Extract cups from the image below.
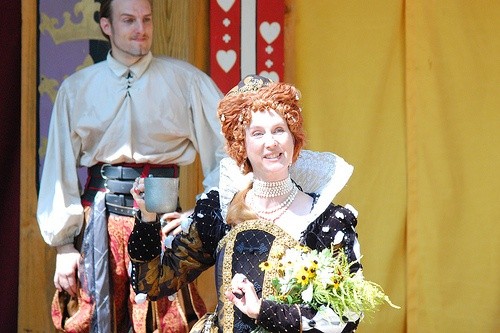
[145,178,179,212]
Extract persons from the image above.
[36,0,228,333]
[128,74,364,333]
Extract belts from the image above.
[86,190,135,207]
[88,164,174,178]
[89,178,135,192]
[106,203,136,216]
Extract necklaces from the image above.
[247,173,299,222]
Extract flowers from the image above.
[259,245,401,325]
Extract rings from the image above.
[242,276,248,283]
[134,176,141,194]
